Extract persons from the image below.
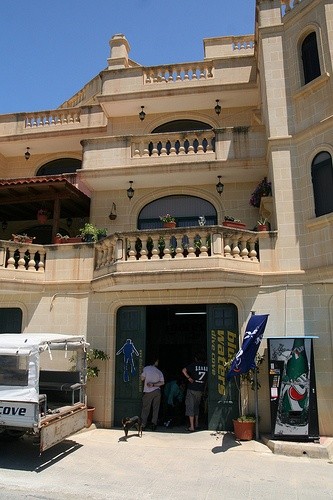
[182,355,208,431]
[141,356,164,430]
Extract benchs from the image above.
[39,381,83,406]
[39,394,46,417]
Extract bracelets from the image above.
[152,384,154,387]
[187,377,191,379]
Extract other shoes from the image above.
[152,425,156,431]
[142,427,145,431]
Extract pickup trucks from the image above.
[0,332,89,454]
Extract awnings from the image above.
[0,178,91,244]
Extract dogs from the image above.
[122,416,144,438]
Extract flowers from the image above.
[249,177,271,208]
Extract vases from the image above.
[109,214,117,220]
[38,215,47,224]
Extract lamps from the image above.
[24,146,31,161]
[66,217,73,227]
[126,181,134,201]
[139,106,146,122]
[215,176,224,197]
[214,99,221,116]
[1,220,8,232]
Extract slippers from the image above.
[185,427,195,432]
[195,426,201,429]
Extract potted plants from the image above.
[158,214,175,222]
[222,215,246,228]
[69,347,111,427]
[224,350,266,441]
[37,208,50,219]
[11,233,36,243]
[256,211,272,231]
[61,234,83,243]
[79,223,95,242]
[95,228,109,240]
[53,233,63,244]
[163,222,176,228]
